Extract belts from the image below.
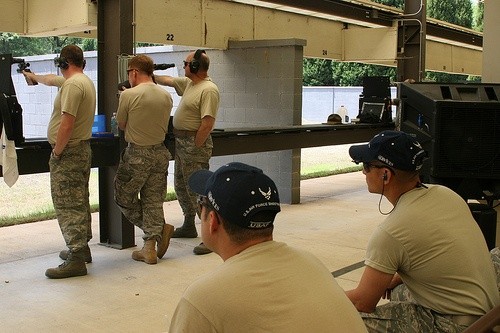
[51,142,82,148]
[442,314,483,327]
[128,142,161,149]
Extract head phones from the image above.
[189,49,206,74]
[54,56,86,69]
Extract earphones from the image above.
[384,173,387,181]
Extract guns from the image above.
[16,62,38,86]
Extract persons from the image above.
[22,45,96,278]
[344,131,500,333]
[153,49,220,254]
[170,162,370,333]
[113,55,174,265]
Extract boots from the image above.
[193,242,213,255]
[45,252,87,279]
[157,224,174,259]
[170,216,198,238]
[59,244,92,264]
[132,240,157,264]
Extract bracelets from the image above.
[53,150,60,158]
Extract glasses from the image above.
[125,68,139,75]
[196,195,222,224]
[182,60,191,67]
[363,162,395,176]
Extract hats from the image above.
[189,162,281,229]
[349,130,425,172]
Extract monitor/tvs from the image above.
[396,82,500,202]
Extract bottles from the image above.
[338,105,351,124]
[110,112,118,136]
